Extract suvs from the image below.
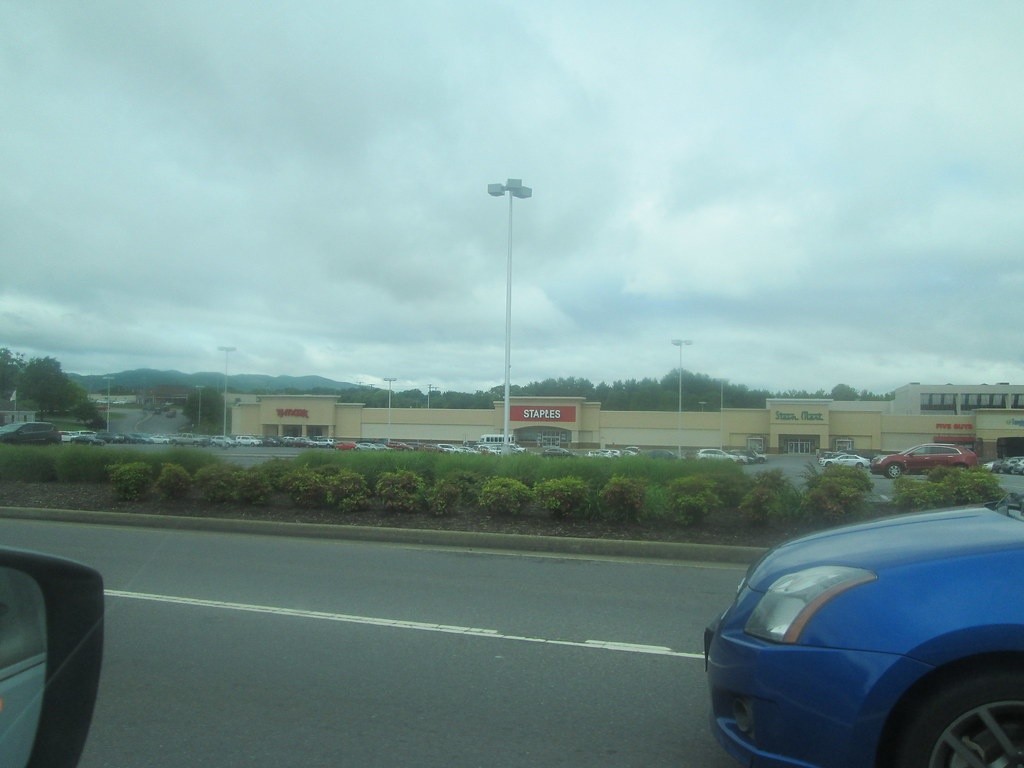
[0,422,62,446]
[869,442,981,479]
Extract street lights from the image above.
[486,177,533,454]
[383,377,397,443]
[102,375,115,435]
[671,339,693,457]
[217,345,236,436]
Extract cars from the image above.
[816,449,871,470]
[61,429,768,465]
[154,404,177,418]
[702,493,1024,768]
[982,455,1024,476]
[96,398,127,406]
